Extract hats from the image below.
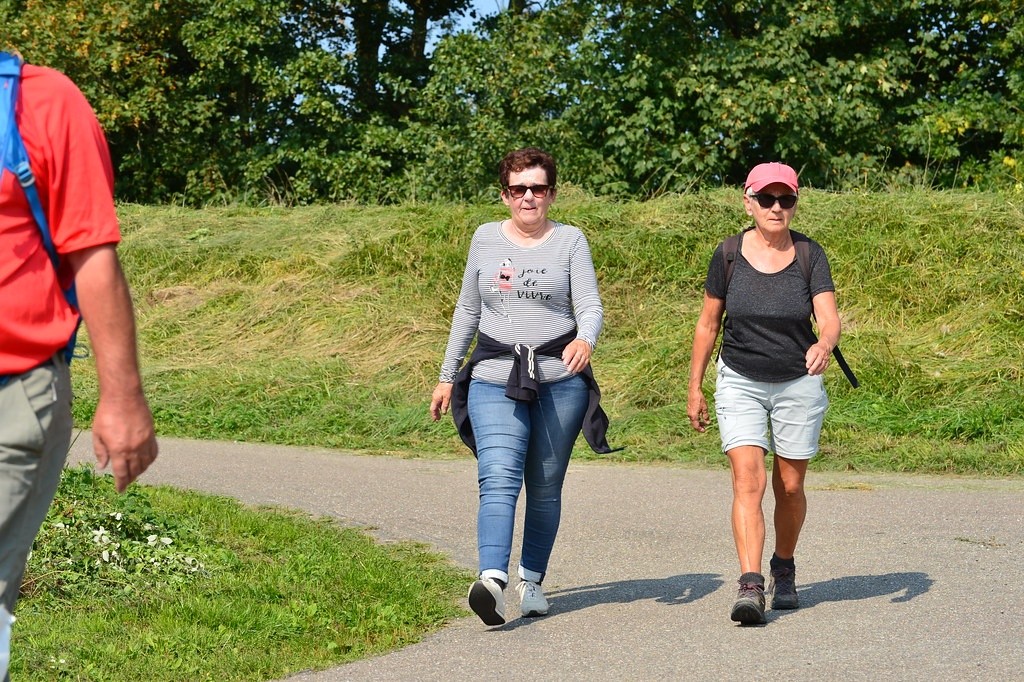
[744,163,799,198]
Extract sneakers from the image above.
[730,580,766,624]
[769,567,800,610]
[466,579,505,627]
[515,580,548,619]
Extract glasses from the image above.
[504,184,552,198]
[749,193,798,208]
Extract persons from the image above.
[431,146,604,627]
[686,162,841,624]
[0,50,157,682]
